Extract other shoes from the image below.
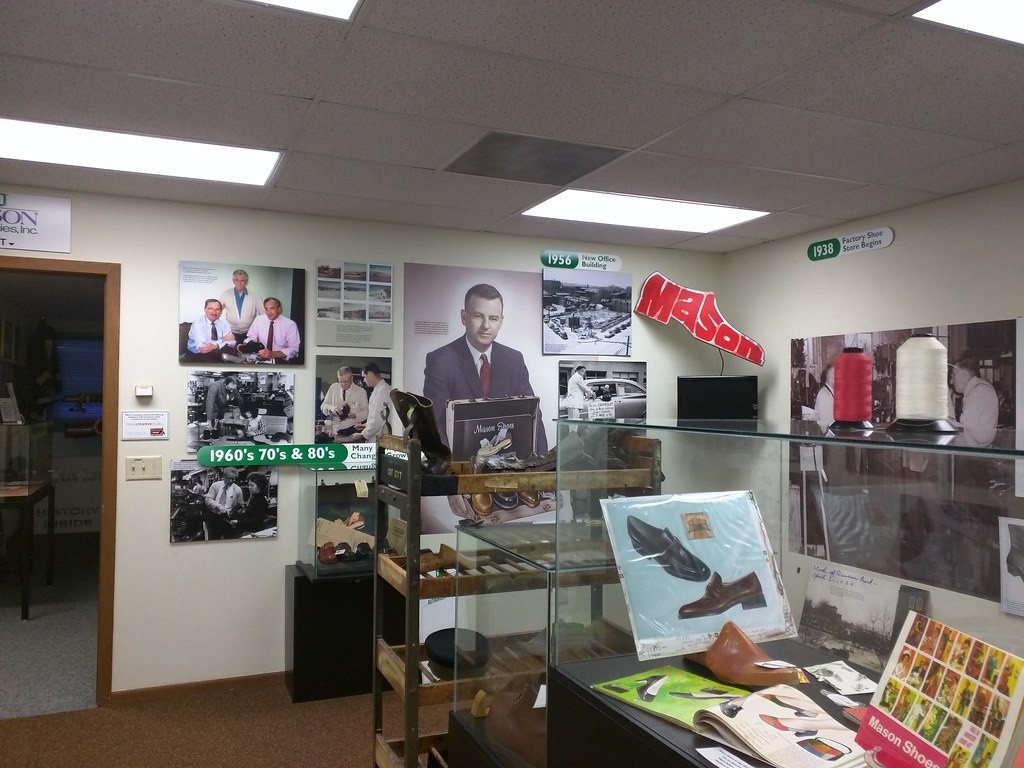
[476,432,513,457]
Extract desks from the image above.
[315,430,365,443]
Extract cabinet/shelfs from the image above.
[372,432,665,767]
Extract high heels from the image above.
[635,675,669,702]
[669,688,753,719]
[755,693,818,719]
[759,714,818,737]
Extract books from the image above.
[590,664,867,768]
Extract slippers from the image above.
[521,451,555,467]
[485,451,527,470]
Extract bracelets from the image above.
[217,343,219,350]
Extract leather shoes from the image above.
[518,490,541,508]
[490,491,521,510]
[318,542,337,563]
[356,542,374,560]
[676,571,767,621]
[335,542,356,562]
[370,537,393,554]
[467,491,494,516]
[626,514,711,582]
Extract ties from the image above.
[479,354,492,397]
[266,321,274,351]
[218,486,227,508]
[210,322,217,341]
[342,389,346,401]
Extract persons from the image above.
[236,297,301,364]
[231,403,267,437]
[351,361,392,443]
[224,472,270,539]
[184,298,236,364]
[597,384,612,401]
[815,361,863,495]
[422,284,549,518]
[949,355,1000,535]
[203,466,245,540]
[193,480,207,495]
[563,366,596,433]
[320,366,368,434]
[200,376,246,430]
[217,270,266,345]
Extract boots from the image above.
[1006,524,1024,582]
[575,424,633,469]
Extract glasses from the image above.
[206,307,221,313]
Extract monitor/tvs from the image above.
[678,375,758,420]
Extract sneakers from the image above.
[334,511,365,530]
